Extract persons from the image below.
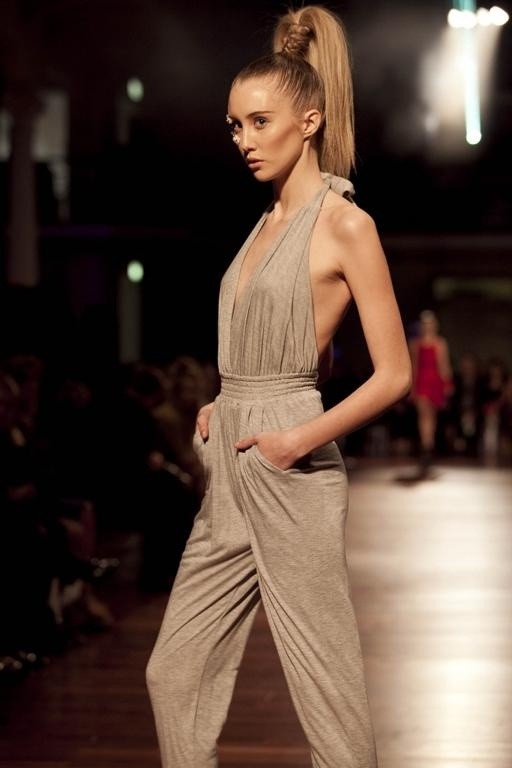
[344,308,511,465]
[1,351,220,672]
[145,5,413,768]
